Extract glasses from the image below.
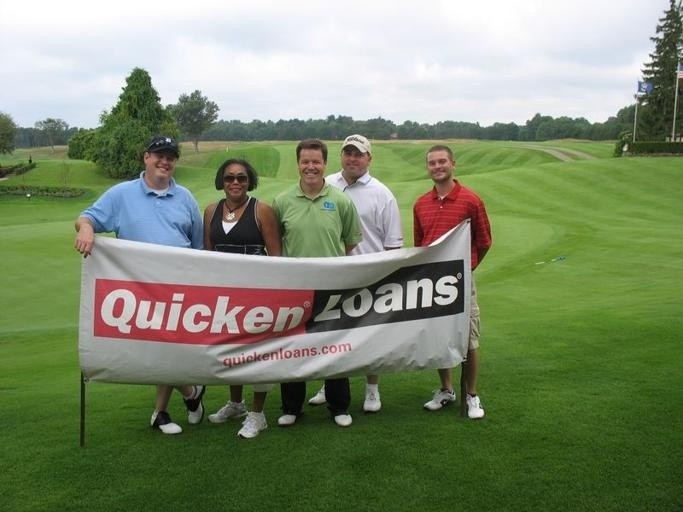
[223,175,247,183]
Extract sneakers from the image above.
[308,384,327,405]
[424,389,456,411]
[334,414,352,426]
[208,399,249,423]
[363,391,381,412]
[466,393,485,419]
[183,384,206,425]
[277,414,297,425]
[150,410,183,434]
[237,410,268,439]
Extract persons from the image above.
[412,144,491,419]
[203,158,281,439]
[307,134,402,413]
[73,136,206,434]
[272,140,362,426]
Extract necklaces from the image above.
[224,196,248,221]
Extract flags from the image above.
[638,81,653,93]
[678,64,683,79]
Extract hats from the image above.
[146,135,178,156]
[340,134,370,155]
